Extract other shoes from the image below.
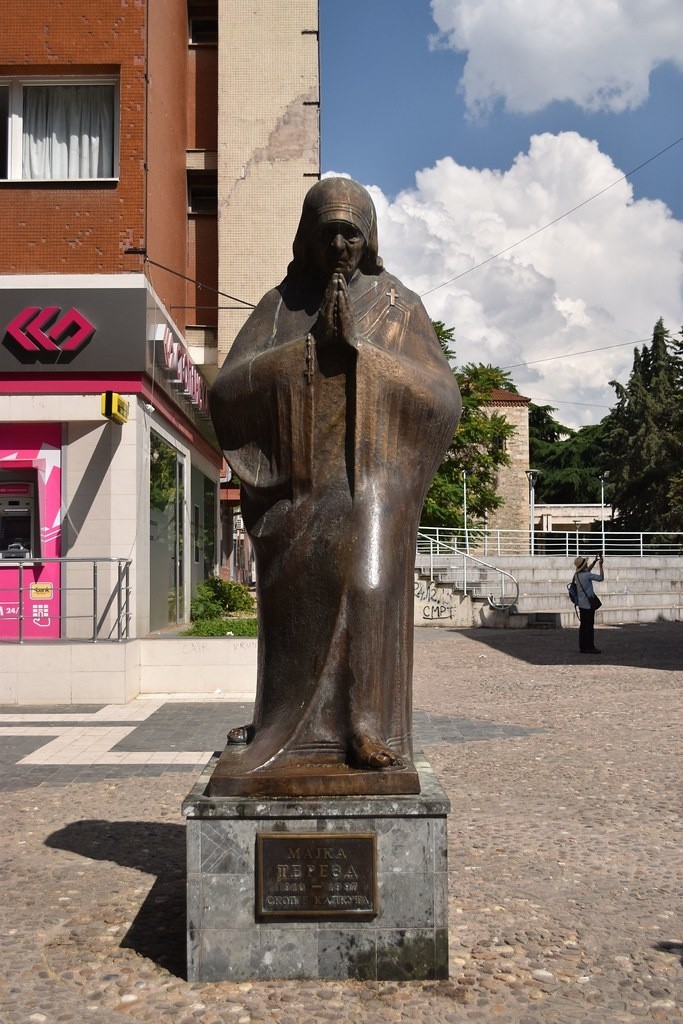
[580,645,601,654]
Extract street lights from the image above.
[524,469,541,556]
[461,469,470,555]
[599,471,611,557]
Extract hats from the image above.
[574,556,589,572]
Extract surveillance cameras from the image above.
[147,405,155,413]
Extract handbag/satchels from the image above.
[577,572,603,610]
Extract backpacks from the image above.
[566,574,578,606]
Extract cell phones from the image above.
[599,553,602,558]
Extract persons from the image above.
[570,552,602,653]
[200,178,462,770]
